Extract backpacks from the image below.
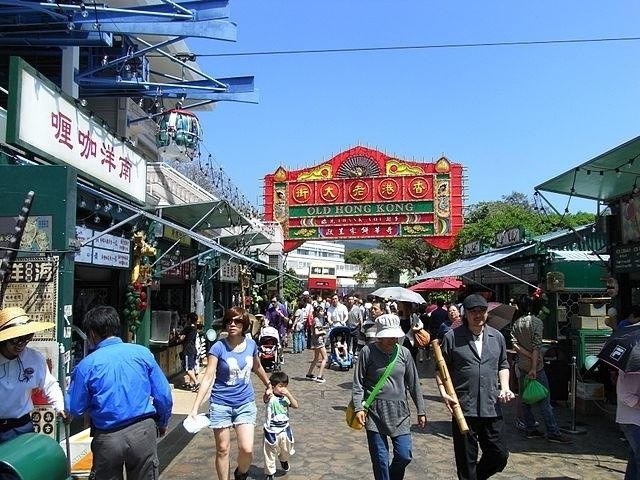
[509,363,519,394]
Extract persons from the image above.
[194,333,202,377]
[352,302,435,366]
[290,290,353,384]
[613,371,640,480]
[264,296,289,365]
[604,308,640,444]
[263,372,298,480]
[508,298,577,445]
[0,306,74,443]
[65,305,172,480]
[272,295,289,348]
[352,314,427,480]
[260,339,276,358]
[188,306,275,480]
[176,310,202,392]
[436,294,518,480]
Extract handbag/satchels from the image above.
[522,376,549,406]
[314,322,327,336]
[347,324,360,338]
[307,314,314,326]
[345,400,368,431]
[413,325,431,347]
[179,350,185,361]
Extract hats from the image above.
[1,306,57,342]
[374,313,405,339]
[463,294,489,309]
[302,290,310,296]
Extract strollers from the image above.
[324,326,352,371]
[259,326,284,371]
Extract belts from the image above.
[1,412,30,432]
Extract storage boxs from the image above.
[597,316,613,330]
[575,398,604,416]
[576,380,605,401]
[576,316,598,330]
[579,303,607,316]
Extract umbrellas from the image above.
[597,326,640,376]
[372,286,427,304]
[449,302,515,332]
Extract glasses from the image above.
[270,300,277,303]
[226,319,246,325]
[8,336,33,344]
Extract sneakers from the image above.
[513,418,539,429]
[526,429,547,437]
[306,374,316,381]
[279,355,284,364]
[192,383,200,391]
[280,461,291,472]
[316,375,326,383]
[265,474,275,480]
[182,384,194,390]
[548,434,573,444]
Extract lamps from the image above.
[116,204,123,214]
[156,85,163,96]
[205,220,212,228]
[564,245,568,251]
[81,6,89,18]
[586,171,592,181]
[533,207,538,215]
[130,68,139,80]
[94,214,101,224]
[100,52,109,67]
[114,71,124,84]
[571,241,578,250]
[591,226,596,233]
[107,199,113,212]
[217,207,223,214]
[569,228,574,234]
[632,185,639,193]
[79,196,86,208]
[130,223,139,233]
[564,209,570,217]
[628,159,634,169]
[89,112,96,125]
[570,188,577,195]
[103,201,108,213]
[125,62,131,72]
[92,22,102,30]
[534,192,539,198]
[587,261,593,267]
[599,171,604,181]
[95,200,102,211]
[175,247,181,256]
[80,95,88,106]
[540,207,545,214]
[138,97,144,109]
[615,167,622,179]
[100,120,107,135]
[152,241,159,247]
[582,235,586,242]
[109,219,116,227]
[154,98,161,107]
[175,97,185,107]
[66,14,76,30]
